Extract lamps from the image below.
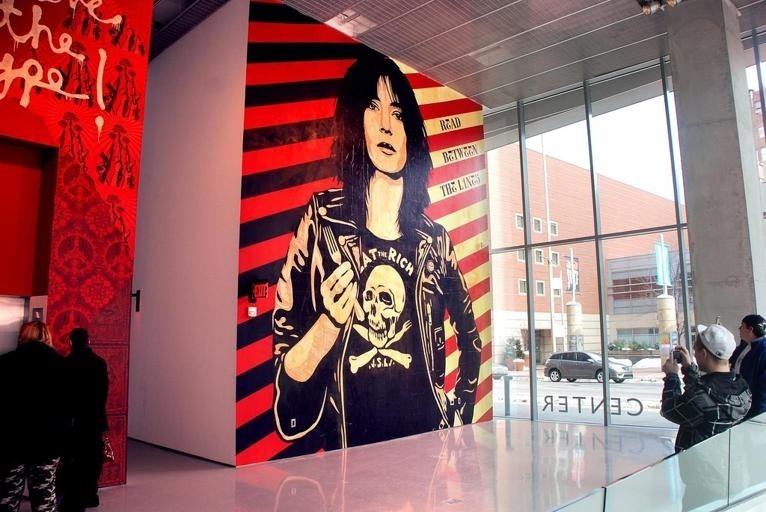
[635,0,683,17]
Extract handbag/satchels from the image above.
[102,437,115,463]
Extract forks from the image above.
[322,226,366,324]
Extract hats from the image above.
[697,324,736,360]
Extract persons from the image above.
[661,325,754,452]
[729,315,766,418]
[56,329,108,512]
[0,320,69,512]
[273,56,481,453]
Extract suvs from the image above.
[542,350,632,384]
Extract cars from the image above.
[492,362,508,381]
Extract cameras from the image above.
[670,350,682,363]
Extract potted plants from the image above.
[511,335,525,372]
[503,334,515,371]
